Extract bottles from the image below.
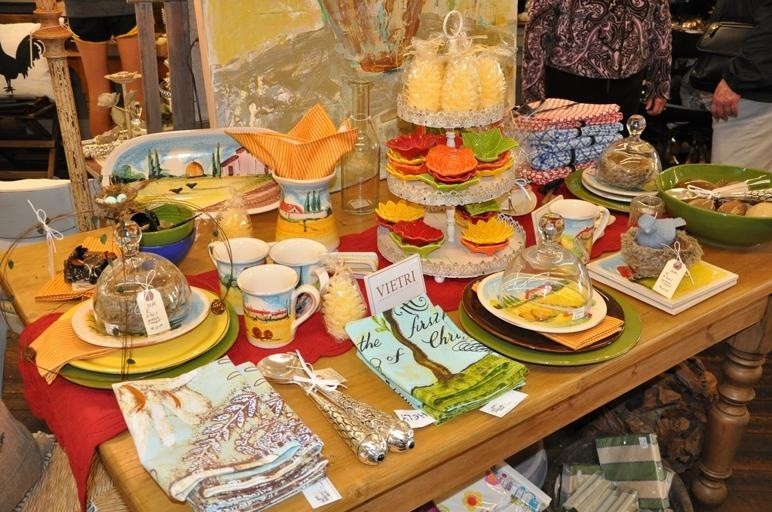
[340,79,380,216]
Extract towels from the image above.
[227,104,360,180]
[515,96,625,186]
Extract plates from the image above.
[510,179,526,213]
[459,270,644,367]
[384,127,520,193]
[564,163,665,213]
[374,200,445,258]
[57,286,240,390]
[454,199,515,256]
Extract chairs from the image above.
[0,0,60,181]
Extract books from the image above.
[430,429,677,512]
[585,250,741,310]
[585,270,739,318]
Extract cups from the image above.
[548,199,610,266]
[499,189,537,216]
[626,195,664,230]
[207,237,331,349]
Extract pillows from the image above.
[0,23,56,103]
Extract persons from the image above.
[707,0,771,176]
[518,0,674,149]
[61,1,149,140]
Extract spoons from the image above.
[258,352,415,465]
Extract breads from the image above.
[676,179,772,217]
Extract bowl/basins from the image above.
[130,203,196,268]
[656,163,772,249]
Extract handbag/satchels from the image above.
[688,21,757,94]
